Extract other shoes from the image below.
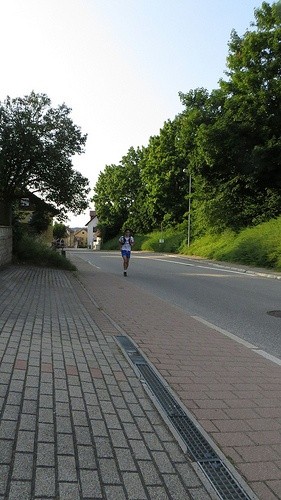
[123,271,127,277]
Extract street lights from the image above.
[183,168,192,248]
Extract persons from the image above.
[119,229,135,276]
